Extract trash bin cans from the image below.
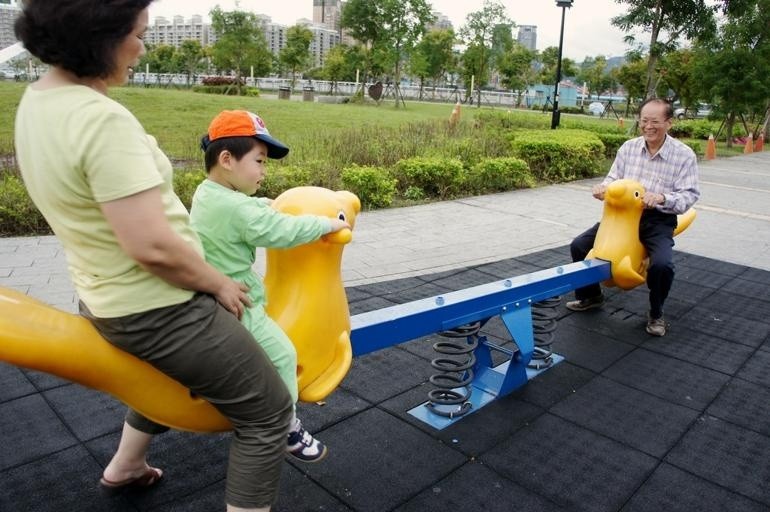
[278,86,291,99]
[303,87,314,101]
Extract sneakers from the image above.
[566,293,606,312]
[645,305,666,337]
[284,417,328,464]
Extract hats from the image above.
[199,109,289,160]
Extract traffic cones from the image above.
[455,101,462,118]
[742,131,754,154]
[754,131,764,152]
[704,133,718,160]
[448,110,459,122]
[618,116,624,128]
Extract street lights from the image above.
[551,0,576,131]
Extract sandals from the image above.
[99,466,164,498]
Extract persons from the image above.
[186,108,352,463]
[9,0,295,511]
[564,97,701,338]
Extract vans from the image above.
[674,102,720,120]
